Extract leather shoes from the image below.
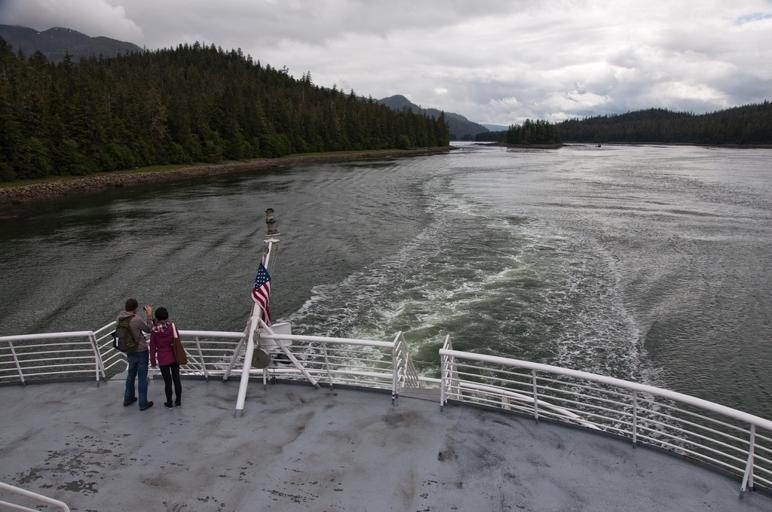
[123,397,137,406]
[140,400,153,411]
[164,402,172,408]
[175,401,180,406]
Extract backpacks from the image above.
[112,315,140,352]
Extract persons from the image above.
[149,306,183,408]
[115,298,156,411]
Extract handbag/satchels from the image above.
[172,337,188,364]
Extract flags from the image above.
[249,264,275,327]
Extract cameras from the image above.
[143,307,146,311]
